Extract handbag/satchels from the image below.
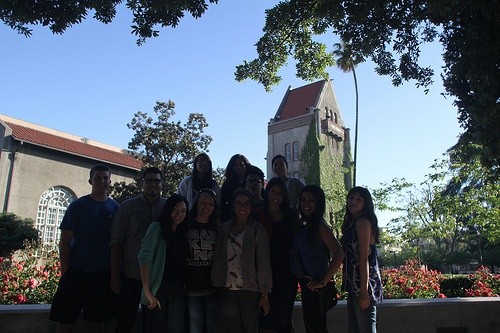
[318,284,337,313]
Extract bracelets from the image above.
[261,295,268,299]
[320,281,326,286]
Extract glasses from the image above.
[246,179,262,184]
[232,200,253,209]
[143,178,162,184]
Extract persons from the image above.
[289,185,346,333]
[174,188,221,333]
[137,195,189,333]
[177,154,220,212]
[210,187,273,333]
[272,155,308,208]
[341,187,382,333]
[252,177,298,333]
[48,164,122,333]
[110,167,168,333]
[220,155,266,222]
